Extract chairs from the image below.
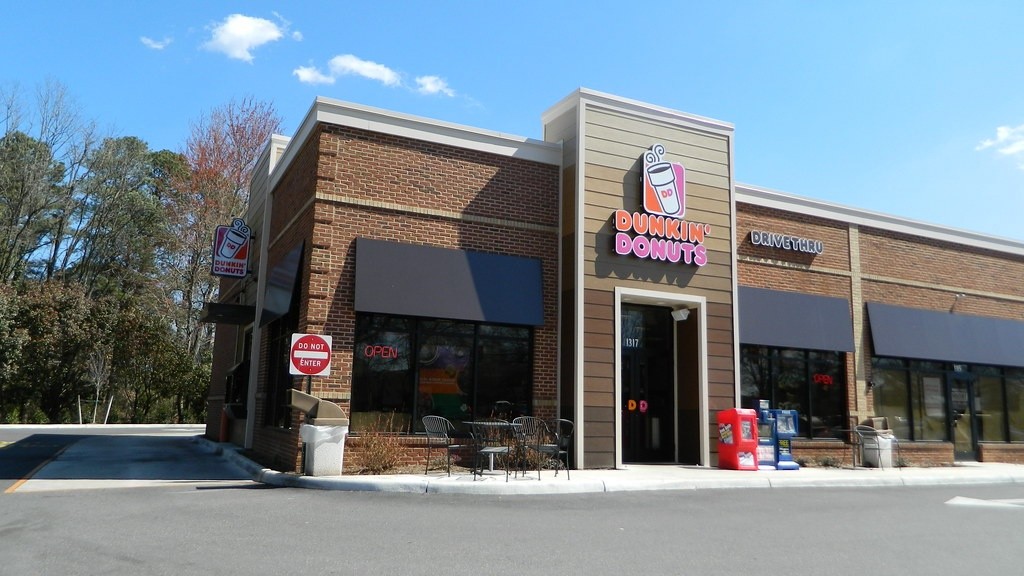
[421,415,476,478]
[522,417,575,481]
[835,414,869,470]
[470,417,521,482]
[512,415,559,477]
[853,425,903,471]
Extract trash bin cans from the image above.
[856,416,894,468]
[286,388,350,476]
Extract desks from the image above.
[461,421,523,476]
[838,428,893,471]
